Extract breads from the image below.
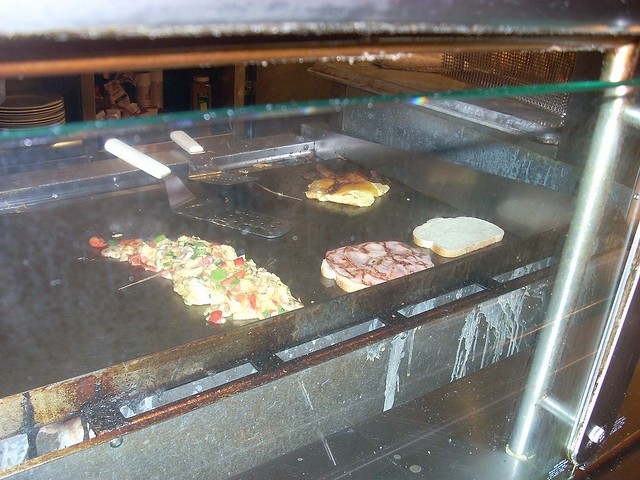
[306,177,389,207]
[413,215,505,258]
[320,240,435,292]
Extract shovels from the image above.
[169,130,261,184]
[104,139,293,240]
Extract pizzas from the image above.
[87,231,305,326]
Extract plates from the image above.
[0,94,64,131]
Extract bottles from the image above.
[190,75,213,111]
[134,72,152,107]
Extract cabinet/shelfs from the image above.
[0,64,245,175]
